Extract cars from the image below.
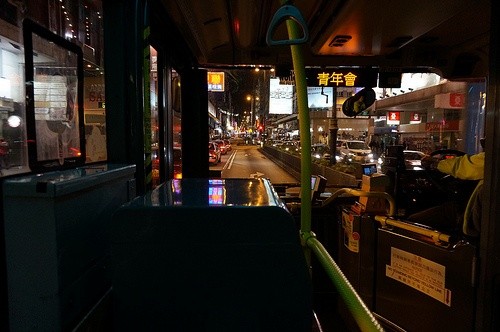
[243,133,427,175]
[148,131,237,179]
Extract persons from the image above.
[420,136,486,248]
[0,137,10,170]
[370,136,445,155]
